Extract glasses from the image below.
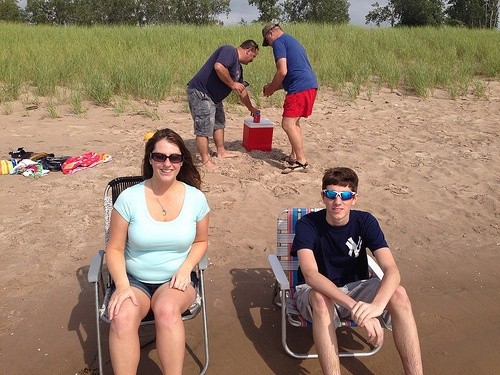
[150,152,184,163]
[323,189,357,201]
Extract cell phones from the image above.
[241,81,249,87]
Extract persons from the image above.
[187,40,260,172]
[262,22,318,174]
[290,166,424,375]
[105,127,211,375]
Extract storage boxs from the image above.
[242,118,274,152]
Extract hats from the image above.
[261,22,279,47]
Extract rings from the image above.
[184,285,187,288]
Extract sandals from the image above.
[282,162,308,174]
[282,155,297,165]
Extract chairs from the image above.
[87,177,209,375]
[268,207,385,359]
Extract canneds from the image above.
[254,110,260,123]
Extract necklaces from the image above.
[151,179,175,215]
